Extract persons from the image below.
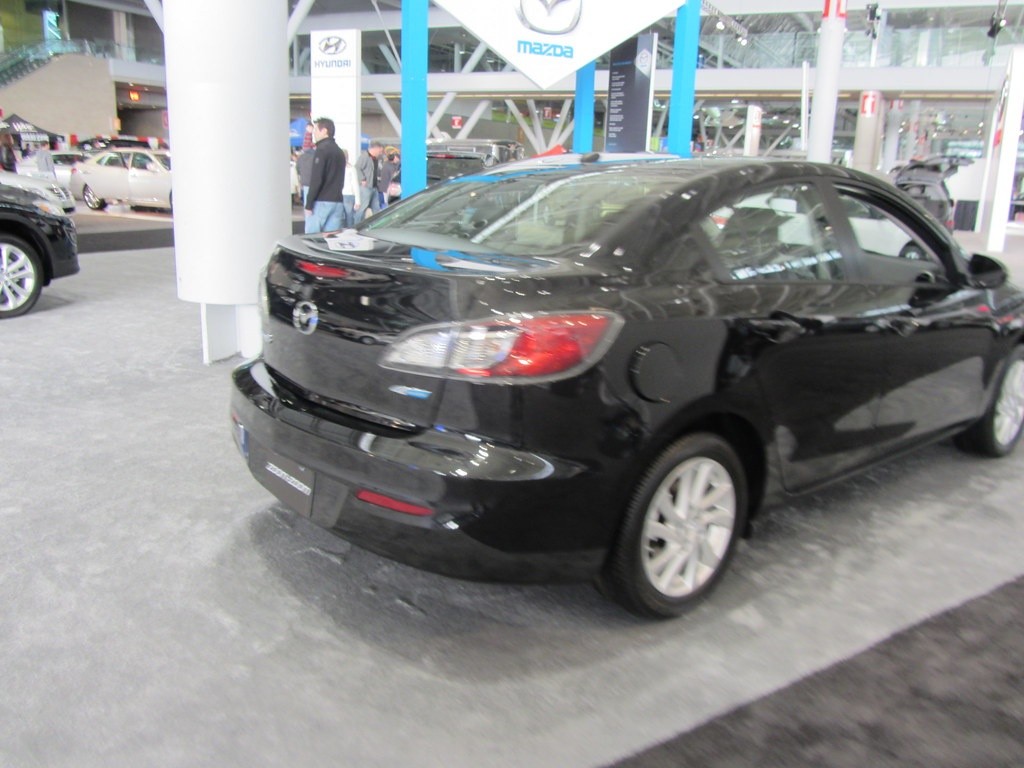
[24,144,30,154]
[380,153,400,205]
[36,140,57,183]
[354,139,382,223]
[304,118,346,234]
[0,129,17,173]
[289,144,316,204]
[341,150,360,227]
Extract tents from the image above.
[290,117,371,149]
[2,114,65,143]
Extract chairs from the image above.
[716,207,816,281]
[516,220,576,254]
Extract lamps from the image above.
[988,16,1004,38]
[866,4,882,38]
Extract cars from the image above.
[0,168,76,214]
[0,180,80,318]
[230,153,1024,619]
[71,147,173,210]
[387,139,524,206]
